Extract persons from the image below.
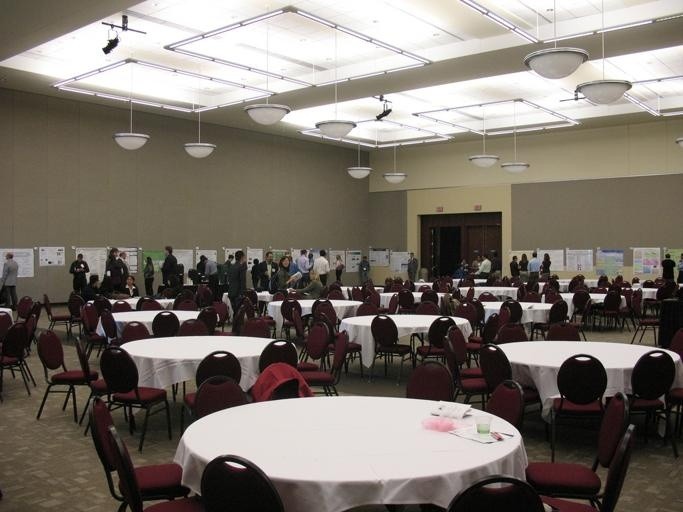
[196,247,344,318]
[359,255,370,286]
[676,253,682,284]
[143,247,179,298]
[1,254,18,311]
[69,247,140,300]
[407,253,418,283]
[457,254,499,280]
[660,253,675,280]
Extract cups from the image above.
[474,415,491,434]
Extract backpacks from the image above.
[268,273,278,294]
